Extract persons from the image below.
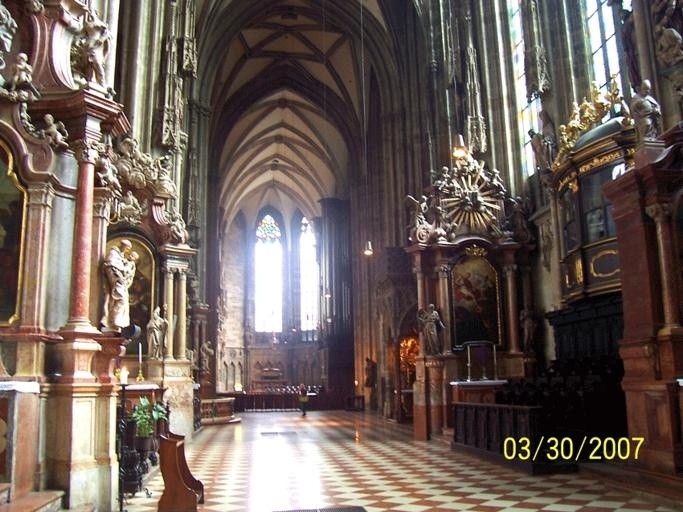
[146,308,170,360]
[81,9,110,87]
[519,303,538,351]
[631,80,664,141]
[298,383,309,416]
[526,82,603,174]
[422,304,447,354]
[10,54,68,152]
[189,277,212,371]
[455,272,490,310]
[100,240,139,332]
[406,156,528,240]
[97,136,188,240]
[653,24,683,69]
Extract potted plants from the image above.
[122,395,168,452]
